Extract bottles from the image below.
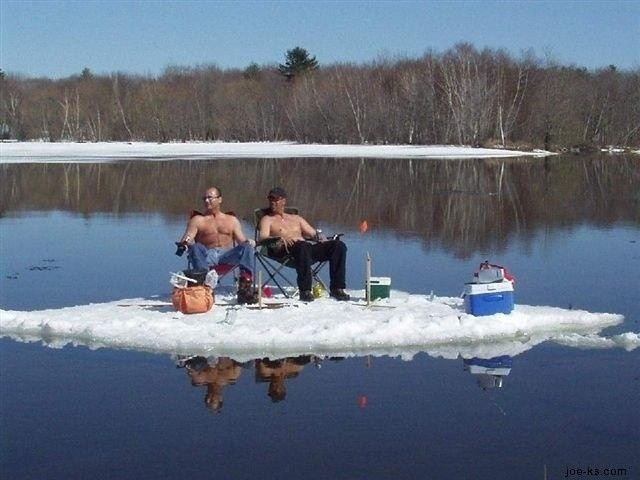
[313,280,323,299]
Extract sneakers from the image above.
[331,287,350,301]
[300,289,315,301]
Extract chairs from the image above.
[185,208,241,306]
[252,207,346,299]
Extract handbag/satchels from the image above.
[173,285,215,315]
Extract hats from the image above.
[267,187,288,199]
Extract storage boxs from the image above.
[464,281,515,317]
[463,352,512,379]
[365,276,392,301]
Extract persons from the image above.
[174,184,260,305]
[183,355,244,416]
[256,187,352,302]
[253,358,305,405]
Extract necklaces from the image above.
[278,215,288,224]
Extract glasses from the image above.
[202,195,219,201]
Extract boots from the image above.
[238,276,258,305]
